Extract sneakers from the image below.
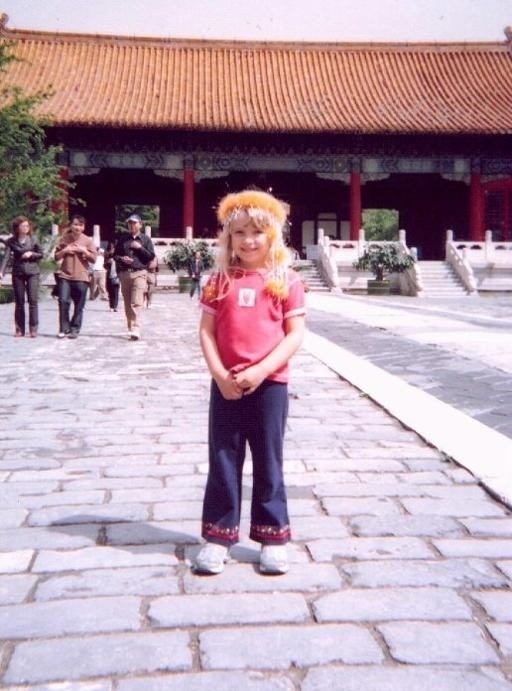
[30,331,37,337]
[260,543,289,574]
[58,333,66,338]
[14,330,24,337]
[190,292,201,300]
[109,308,117,312]
[68,331,78,338]
[127,331,140,340]
[142,300,152,309]
[195,542,231,573]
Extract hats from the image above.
[126,215,141,222]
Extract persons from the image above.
[194,190,306,577]
[49,235,159,312]
[188,250,204,299]
[0,216,43,337]
[55,214,97,339]
[113,215,156,342]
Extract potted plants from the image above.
[354,246,414,295]
[162,241,214,293]
